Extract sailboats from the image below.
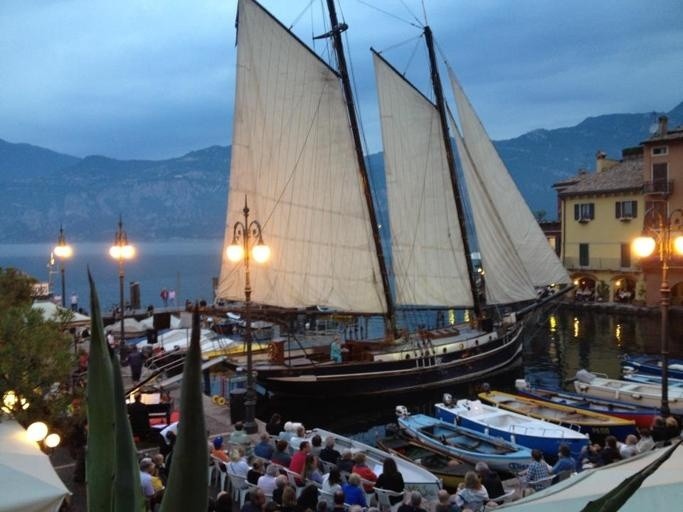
[215,0,578,404]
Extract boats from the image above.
[285,354,682,499]
[0,266,54,303]
[279,312,349,347]
[106,304,287,370]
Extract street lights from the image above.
[53,224,73,309]
[226,192,271,435]
[68,327,90,357]
[108,212,135,366]
[629,206,682,441]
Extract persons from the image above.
[524,428,656,494]
[138,413,405,511]
[53,285,208,387]
[576,284,637,304]
[128,392,151,449]
[330,333,350,363]
[647,415,682,442]
[398,461,505,511]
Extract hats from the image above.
[214,436,223,445]
